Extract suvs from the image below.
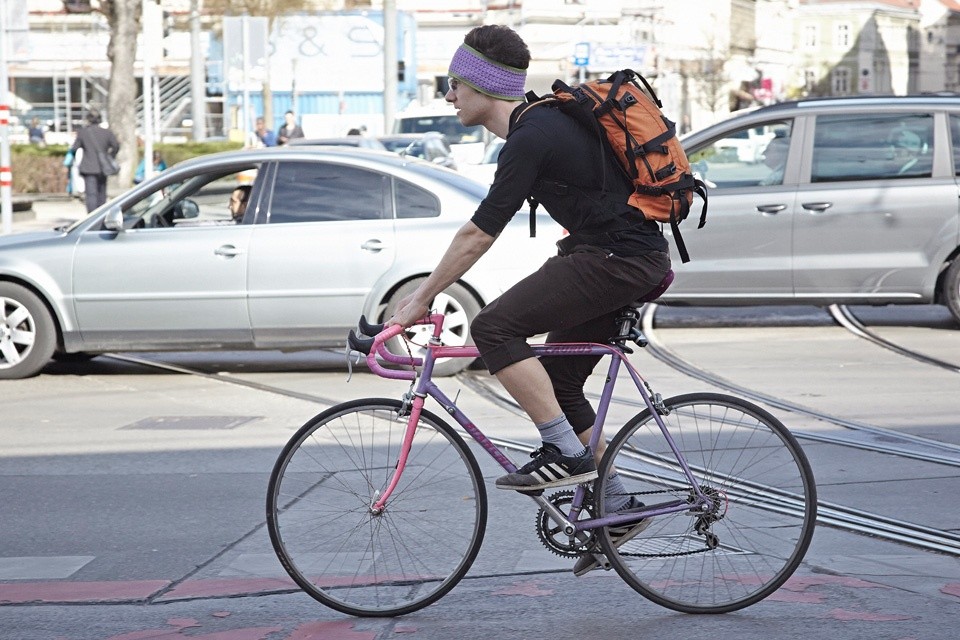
[651,90,960,326]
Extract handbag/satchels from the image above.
[96,152,120,177]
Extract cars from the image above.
[0,143,569,381]
[284,98,551,218]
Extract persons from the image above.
[228,185,252,224]
[890,129,926,174]
[132,150,166,184]
[346,129,387,151]
[382,24,671,578]
[758,136,788,186]
[28,117,44,144]
[70,109,120,214]
[253,117,276,147]
[729,80,754,112]
[277,111,304,145]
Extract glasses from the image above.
[447,77,465,93]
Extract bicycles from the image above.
[263,269,822,617]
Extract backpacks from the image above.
[517,66,707,264]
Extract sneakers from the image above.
[494,440,599,490]
[571,497,657,576]
